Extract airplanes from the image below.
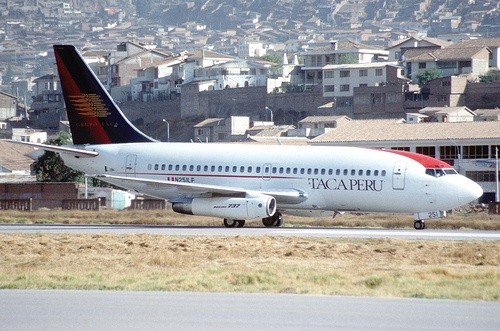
[0,43,486,231]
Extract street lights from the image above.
[265,106,273,121]
[161,119,171,142]
